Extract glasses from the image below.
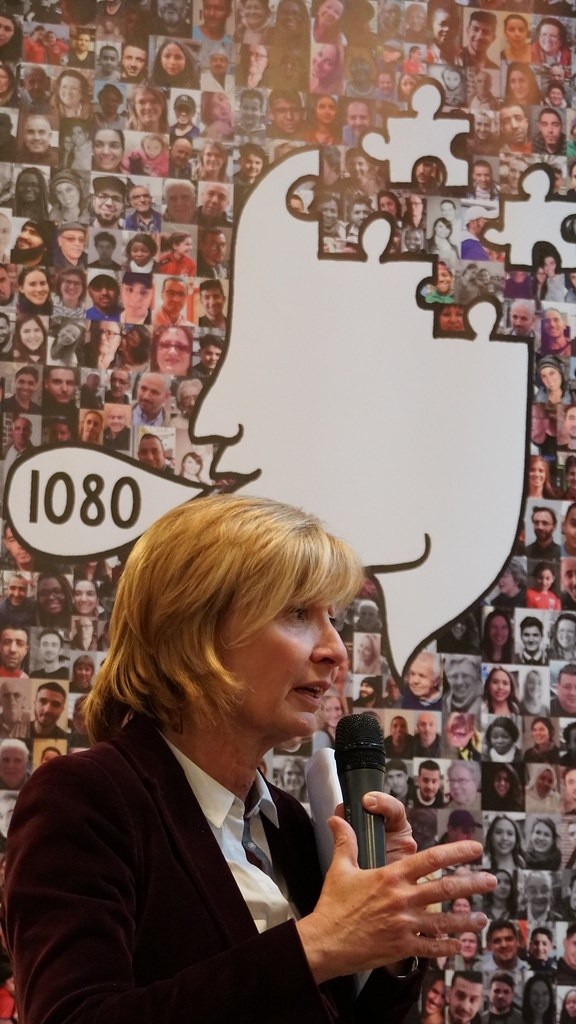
[93,328,121,339]
[62,235,84,243]
[245,51,265,58]
[3,693,24,699]
[159,342,191,354]
[446,777,474,784]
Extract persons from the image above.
[1,495,495,1024]
[1,0,575,1024]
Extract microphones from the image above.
[333,715,388,872]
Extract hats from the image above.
[122,272,153,288]
[92,175,126,199]
[59,222,86,234]
[449,811,482,830]
[536,354,564,378]
[464,207,499,225]
[361,676,379,693]
[21,218,55,248]
[52,168,82,191]
[173,95,196,111]
